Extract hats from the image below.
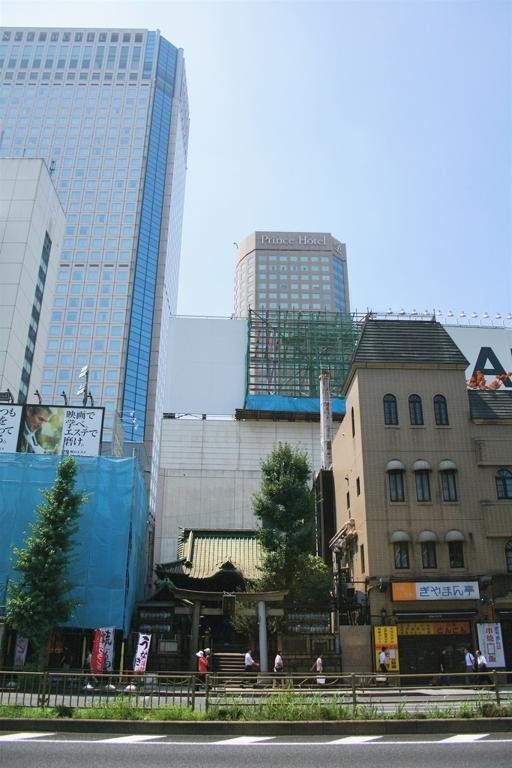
[204,648,210,652]
[196,651,204,658]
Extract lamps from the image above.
[384,305,512,327]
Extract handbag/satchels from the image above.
[276,665,282,672]
[472,660,478,672]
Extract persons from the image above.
[274,650,284,685]
[316,653,327,683]
[463,646,475,687]
[18,405,58,452]
[379,646,390,679]
[438,648,450,684]
[59,645,74,672]
[204,648,215,689]
[474,649,495,688]
[83,648,98,685]
[239,650,260,687]
[196,650,209,689]
